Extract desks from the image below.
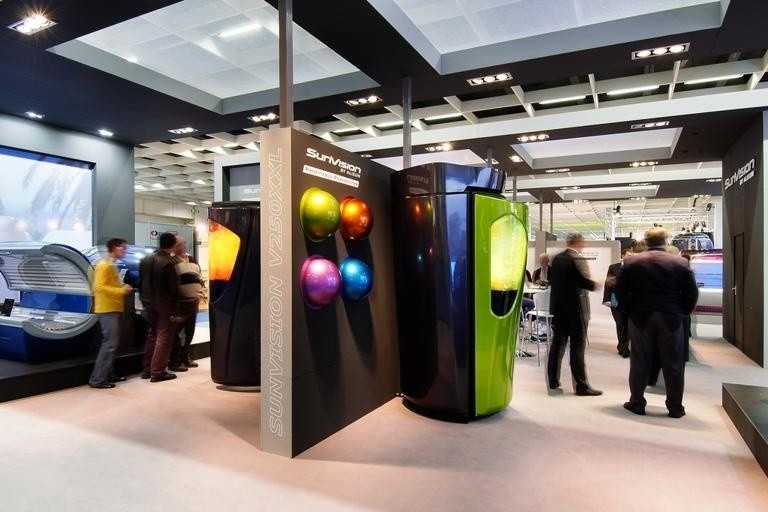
[523,286,546,343]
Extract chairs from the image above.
[520,288,555,366]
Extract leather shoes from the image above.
[91,357,198,388]
[573,387,603,397]
[618,348,685,418]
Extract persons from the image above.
[88,238,133,390]
[138,232,177,383]
[168,236,202,372]
[520,226,699,418]
